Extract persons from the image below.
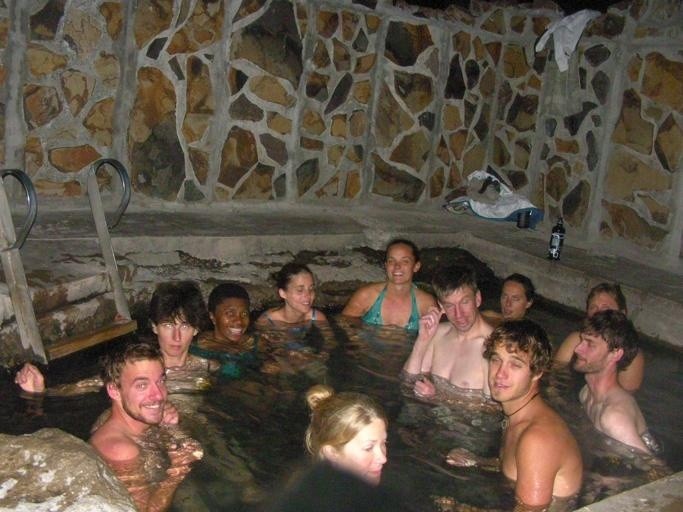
[192,285,309,382]
[336,240,438,376]
[481,271,536,325]
[302,384,389,489]
[546,283,645,403]
[251,265,339,374]
[13,279,231,435]
[571,309,673,492]
[393,257,503,414]
[86,334,204,511]
[431,318,583,511]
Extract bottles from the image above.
[544,216,566,261]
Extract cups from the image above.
[516,211,530,230]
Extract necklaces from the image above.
[501,391,540,430]
